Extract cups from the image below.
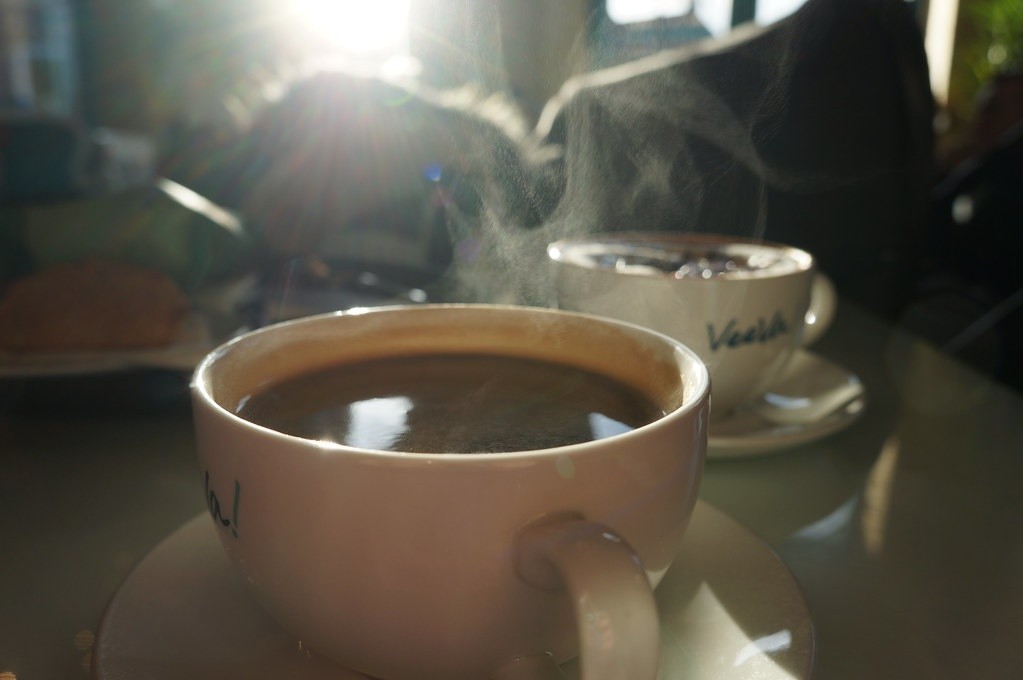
[188,304,715,680]
[544,233,837,422]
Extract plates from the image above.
[93,481,816,680]
[705,350,869,461]
